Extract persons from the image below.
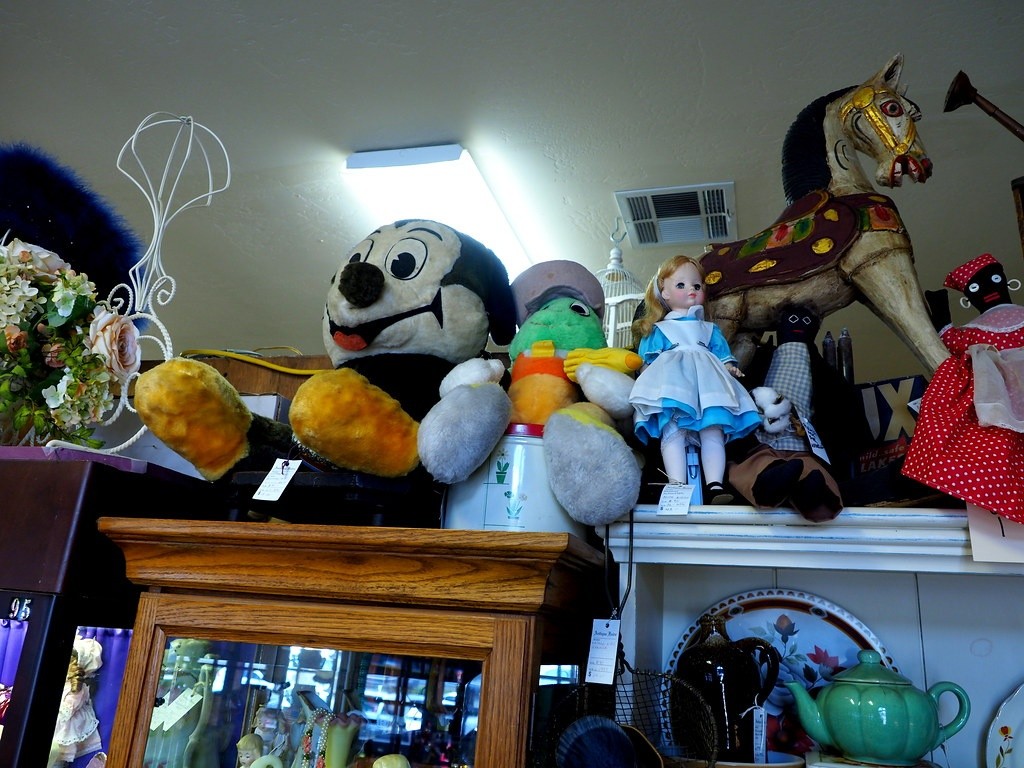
[253,707,286,756]
[628,254,762,505]
[235,734,263,768]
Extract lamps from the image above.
[345,141,535,284]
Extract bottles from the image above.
[671,616,779,764]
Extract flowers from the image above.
[0,227,142,450]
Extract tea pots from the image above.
[783,650,971,768]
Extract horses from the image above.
[631,52,952,375]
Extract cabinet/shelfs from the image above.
[101,514,616,768]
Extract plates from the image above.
[674,751,805,768]
[986,684,1024,768]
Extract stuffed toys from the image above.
[900,253,1024,523]
[415,260,644,527]
[725,303,861,523]
[134,219,515,527]
